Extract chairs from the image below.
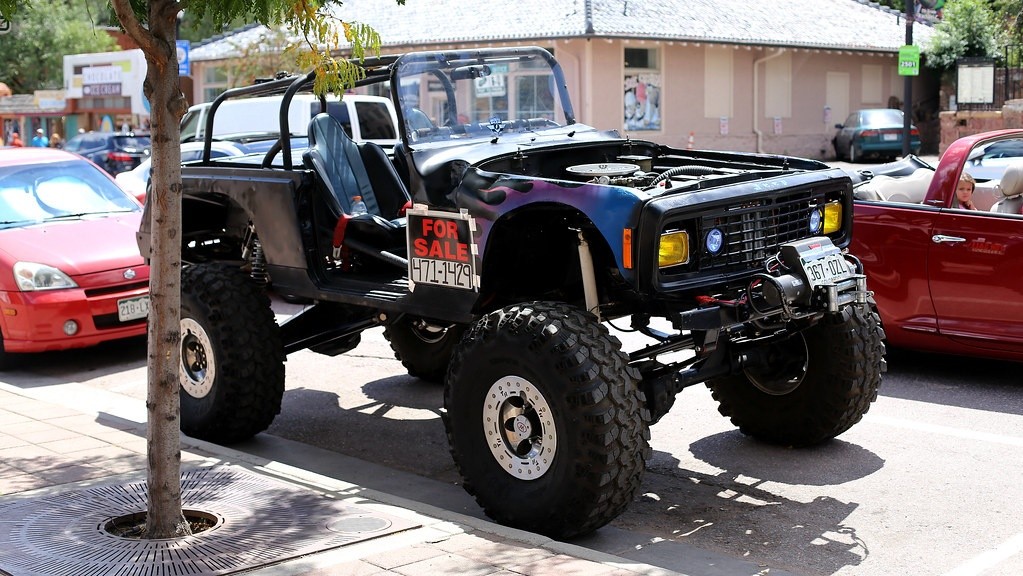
[990,166,1023,214]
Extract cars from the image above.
[831,109,922,164]
[932,137,1023,180]
[0,145,150,371]
[62,94,433,196]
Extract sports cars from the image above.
[841,129,1023,374]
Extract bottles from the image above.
[350,196,367,216]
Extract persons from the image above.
[9,128,63,149]
[956,172,977,210]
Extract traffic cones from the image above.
[686,131,695,149]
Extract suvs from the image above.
[134,46,889,543]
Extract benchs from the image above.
[857,166,935,204]
[973,179,1005,210]
